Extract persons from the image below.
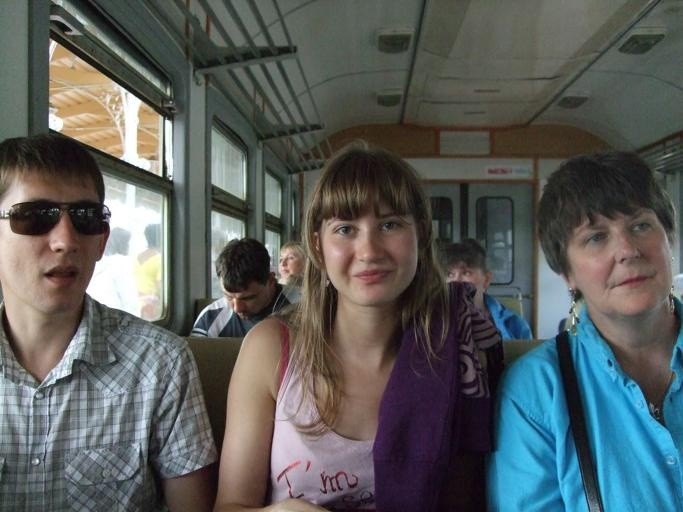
[0,131,219,512]
[104,224,164,323]
[277,240,307,287]
[190,238,291,338]
[214,148,502,512]
[488,151,682,512]
[440,238,533,342]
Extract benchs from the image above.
[189,296,546,450]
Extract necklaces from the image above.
[644,369,673,423]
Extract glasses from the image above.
[0,200,111,235]
